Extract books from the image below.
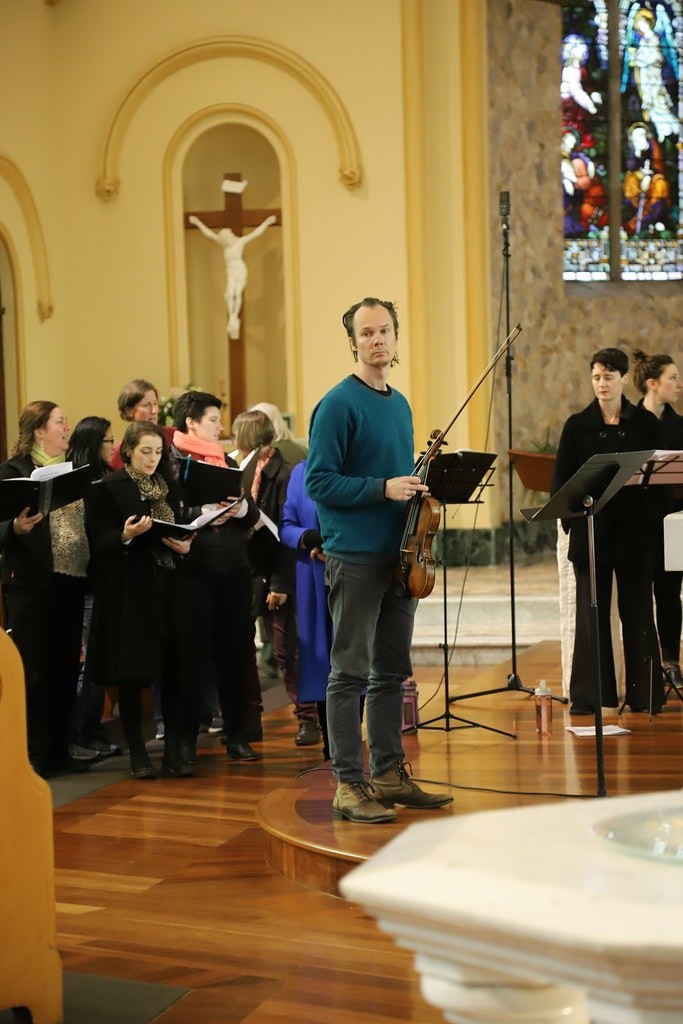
[416,449,498,503]
[0,461,91,515]
[134,500,239,542]
[175,455,242,506]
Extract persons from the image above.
[0,378,366,779]
[189,214,278,340]
[303,296,455,823]
[551,349,683,713]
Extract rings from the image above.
[412,491,416,495]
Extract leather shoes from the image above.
[331,780,398,823]
[295,723,320,745]
[178,754,198,778]
[157,758,178,778]
[369,758,454,809]
[225,738,261,762]
[131,765,155,778]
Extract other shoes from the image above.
[568,702,595,715]
[665,665,683,689]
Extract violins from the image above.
[397,429,447,599]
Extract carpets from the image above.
[1,968,191,1024]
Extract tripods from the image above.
[401,503,517,739]
[446,216,569,704]
[618,485,683,722]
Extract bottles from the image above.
[399,678,419,735]
[535,680,553,737]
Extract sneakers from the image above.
[153,721,165,739]
[208,720,223,733]
[68,743,101,760]
[86,737,123,758]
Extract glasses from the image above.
[101,436,114,445]
